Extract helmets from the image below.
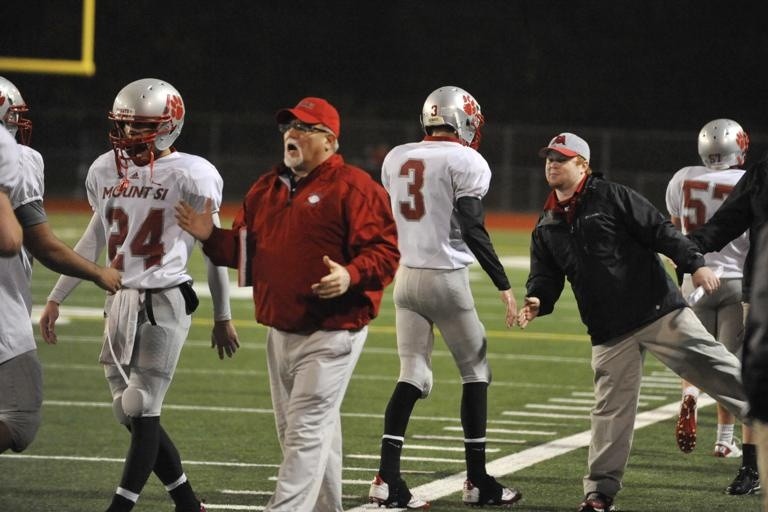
[107,78,185,191]
[697,118,750,170]
[0,76,32,146]
[420,86,486,151]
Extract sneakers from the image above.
[368,474,431,510]
[712,435,743,459]
[577,492,617,511]
[462,475,523,508]
[674,394,696,455]
[725,466,761,497]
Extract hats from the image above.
[275,96,341,141]
[537,133,591,167]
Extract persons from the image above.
[0,124,25,257]
[0,78,122,453]
[371,85,520,512]
[174,97,398,512]
[676,116,767,498]
[38,77,238,512]
[664,119,744,461]
[513,131,750,510]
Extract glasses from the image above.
[277,124,330,136]
